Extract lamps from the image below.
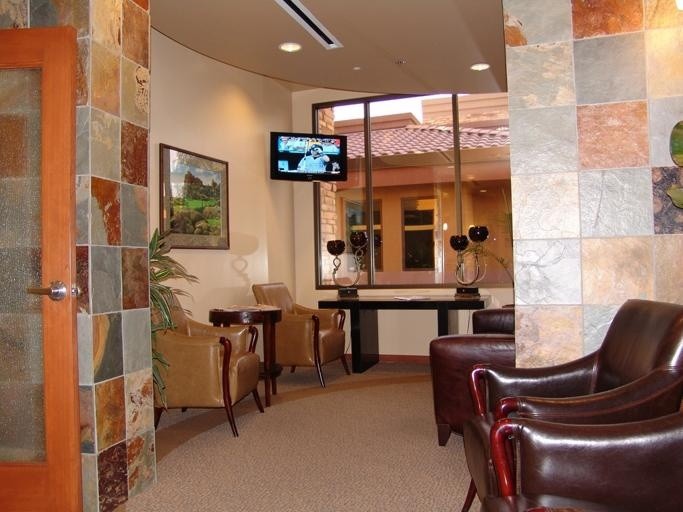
[449,225,490,301]
[326,230,370,301]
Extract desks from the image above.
[317,295,490,374]
[208,303,284,407]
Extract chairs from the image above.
[481,408,683,511]
[429,307,515,448]
[451,297,683,512]
[148,285,264,438]
[253,281,352,396]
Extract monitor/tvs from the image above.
[271,132,347,181]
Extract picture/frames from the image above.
[158,143,231,251]
